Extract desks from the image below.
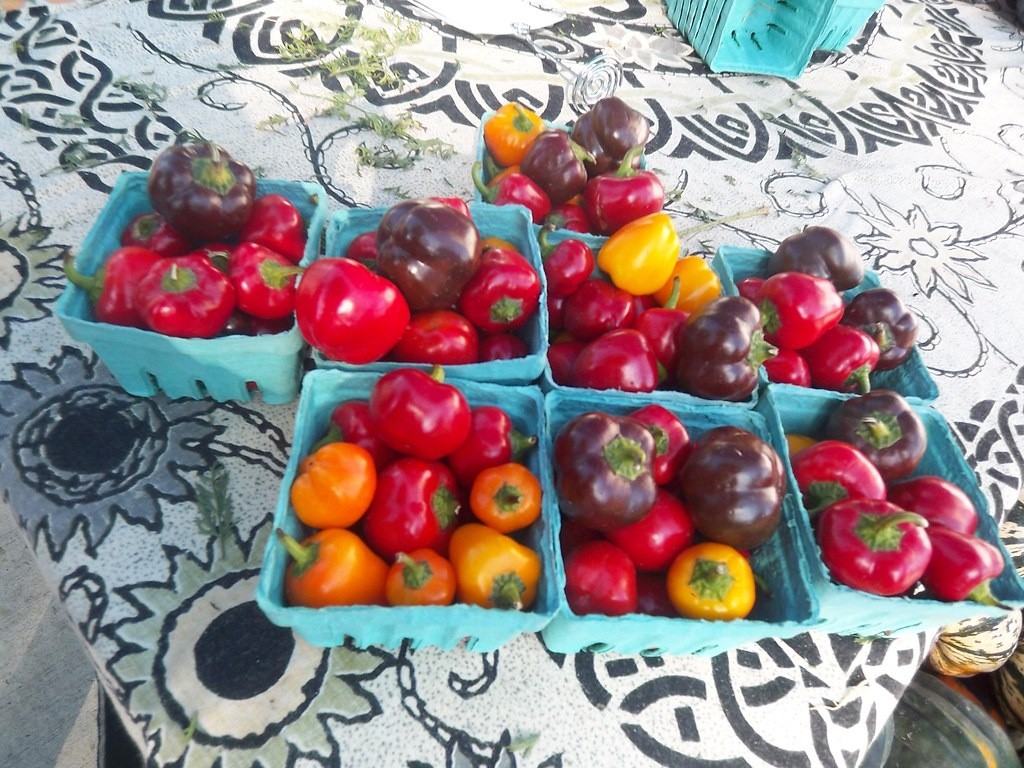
[0,0,1024,768]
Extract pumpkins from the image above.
[851,498,1024,768]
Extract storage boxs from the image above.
[53,0,1023,654]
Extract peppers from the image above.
[277,362,541,611]
[64,139,306,340]
[783,390,1013,611]
[471,96,664,235]
[535,212,780,403]
[739,225,917,394]
[295,193,541,367]
[551,403,786,621]
